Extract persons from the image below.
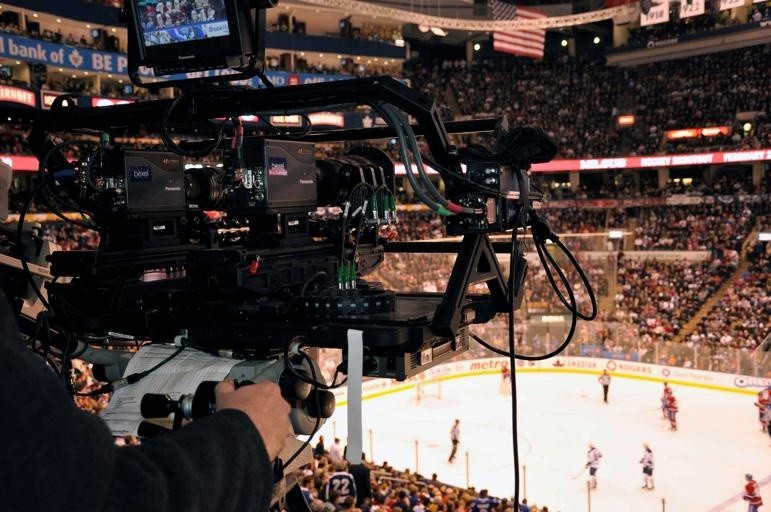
[0,1,770,374]
[598,367,612,403]
[743,473,763,512]
[1,220,557,512]
[660,381,679,431]
[582,439,602,491]
[640,442,655,489]
[754,386,771,436]
